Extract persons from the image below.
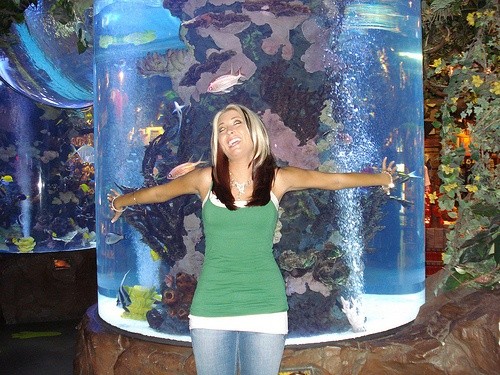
[108,103,396,375]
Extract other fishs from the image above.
[94,0,423,321]
[0,78,96,253]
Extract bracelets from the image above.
[126,188,137,210]
[380,170,393,191]
[108,192,129,214]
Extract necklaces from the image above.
[226,161,258,200]
